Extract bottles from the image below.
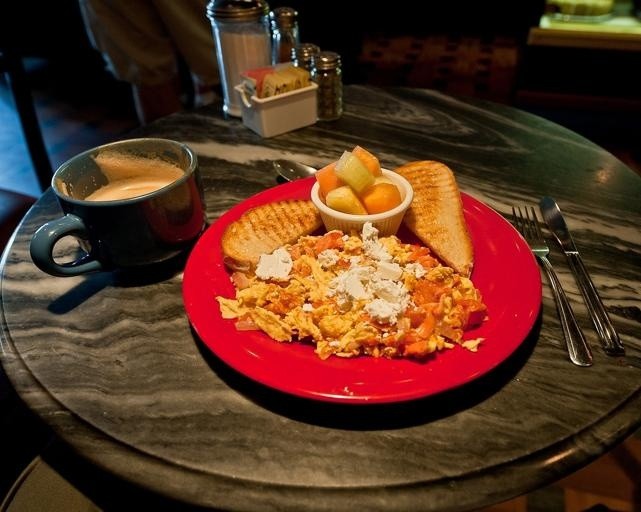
[205,0,272,118]
[269,7,301,66]
[290,44,321,72]
[311,51,342,122]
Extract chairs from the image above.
[0,48,54,255]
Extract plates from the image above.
[182,175,542,407]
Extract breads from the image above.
[395,158,476,278]
[219,198,323,274]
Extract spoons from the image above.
[272,159,317,181]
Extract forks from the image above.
[512,205,593,368]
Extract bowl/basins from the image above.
[310,168,414,237]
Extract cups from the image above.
[29,137,207,274]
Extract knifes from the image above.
[538,197,627,358]
